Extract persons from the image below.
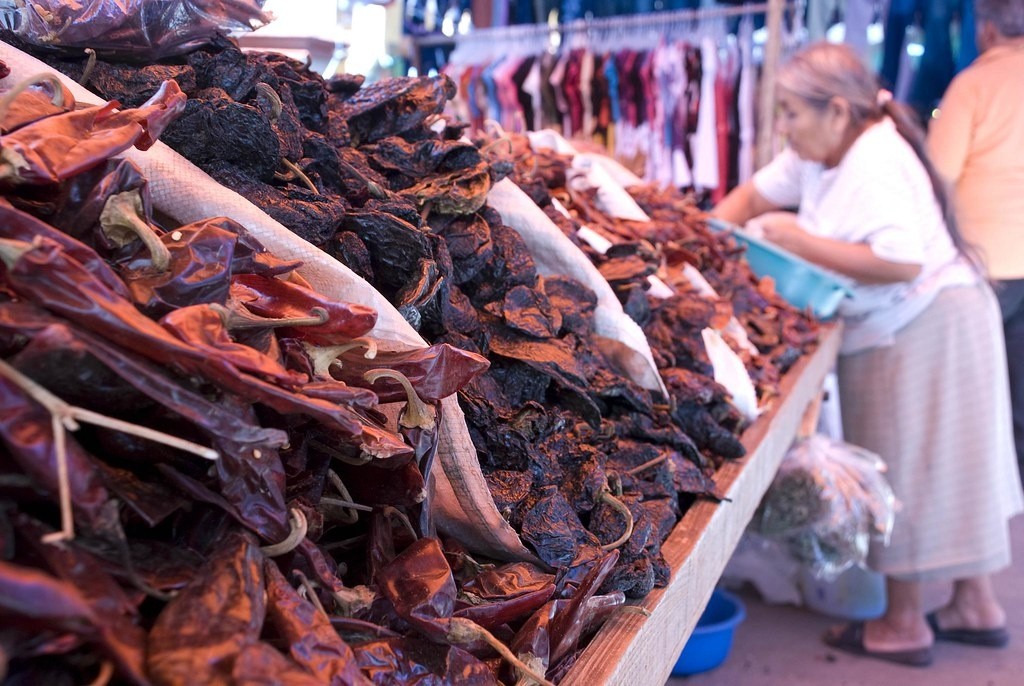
[708,43,1023,664]
[926,0,1024,487]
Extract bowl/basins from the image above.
[673,592,747,676]
[704,216,853,318]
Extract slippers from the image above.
[822,620,933,666]
[926,611,1008,646]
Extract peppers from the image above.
[0,45,747,686]
[474,123,824,355]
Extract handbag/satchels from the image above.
[747,429,900,570]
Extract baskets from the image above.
[704,217,853,320]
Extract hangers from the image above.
[445,3,756,64]
